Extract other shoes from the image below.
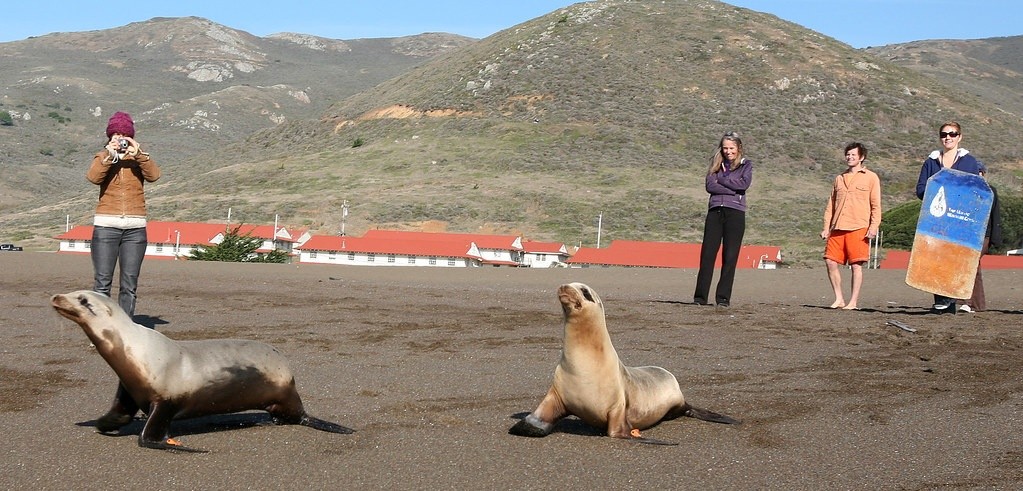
[934,304,949,309]
[960,305,972,313]
[694,298,707,305]
[718,302,730,307]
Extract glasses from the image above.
[940,131,960,138]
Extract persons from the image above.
[692,130,752,306]
[916,121,1000,312]
[820,143,882,309]
[86,111,160,317]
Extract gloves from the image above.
[735,189,745,195]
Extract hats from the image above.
[976,160,986,173]
[106,111,134,139]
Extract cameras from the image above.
[115,139,129,149]
